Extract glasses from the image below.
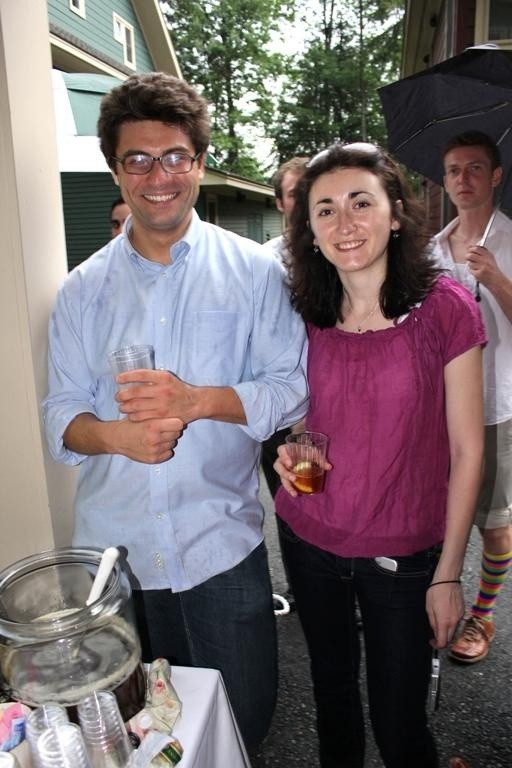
[305,142,379,170]
[113,150,202,176]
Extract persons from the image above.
[262,155,314,612]
[109,198,134,239]
[420,131,512,664]
[273,143,486,767]
[41,69,312,765]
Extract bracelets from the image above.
[428,579,461,591]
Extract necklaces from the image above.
[344,298,379,334]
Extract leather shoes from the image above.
[448,615,494,662]
[274,590,298,613]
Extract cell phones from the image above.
[429,658,441,713]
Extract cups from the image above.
[0,547,146,729]
[23,690,137,767]
[107,342,155,396]
[285,429,331,498]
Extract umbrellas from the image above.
[376,41,510,249]
